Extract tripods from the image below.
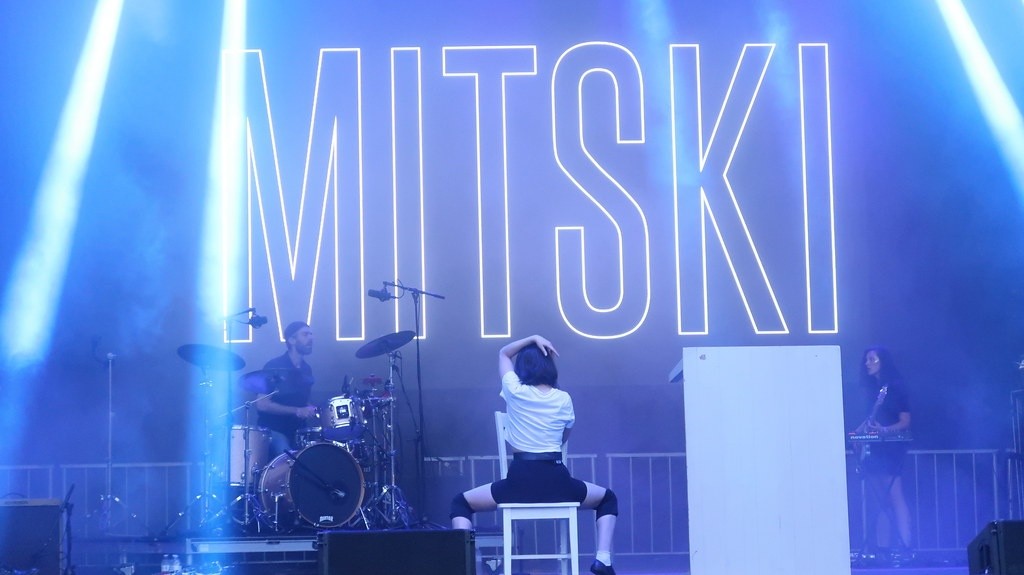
[345,281,452,532]
[65,306,285,540]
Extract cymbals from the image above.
[234,368,283,392]
[355,329,415,358]
[176,344,246,372]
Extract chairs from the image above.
[495,411,581,575]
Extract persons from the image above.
[853,348,912,568]
[450,334,618,575]
[256,322,317,535]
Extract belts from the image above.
[514,452,563,461]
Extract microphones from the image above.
[368,289,392,302]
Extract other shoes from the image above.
[590,559,618,575]
[286,511,300,528]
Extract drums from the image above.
[214,422,272,488]
[296,427,326,446]
[258,438,367,529]
[318,395,366,438]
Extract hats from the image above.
[283,321,309,340]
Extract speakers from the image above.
[0,498,69,575]
[312,527,478,575]
